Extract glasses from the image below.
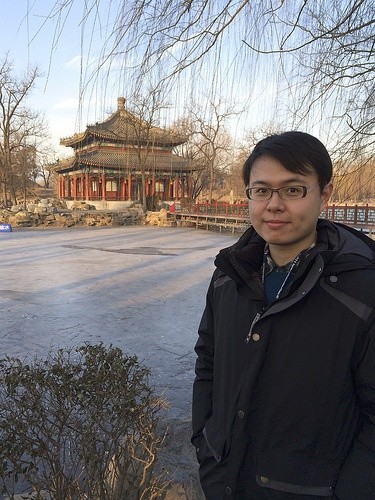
[245,184,321,199]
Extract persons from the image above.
[188,131,375,500]
